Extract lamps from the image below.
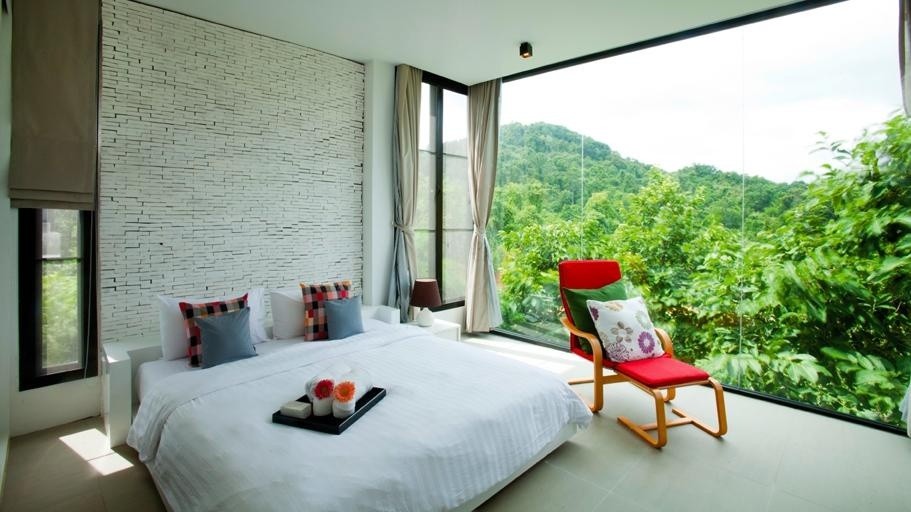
[520,41,532,58]
[410,278,442,327]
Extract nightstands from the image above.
[407,318,461,342]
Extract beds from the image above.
[101,305,577,512]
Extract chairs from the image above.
[559,258,727,449]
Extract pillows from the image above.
[585,295,665,363]
[270,280,367,341]
[561,277,628,353]
[157,287,270,369]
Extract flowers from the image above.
[313,378,356,403]
